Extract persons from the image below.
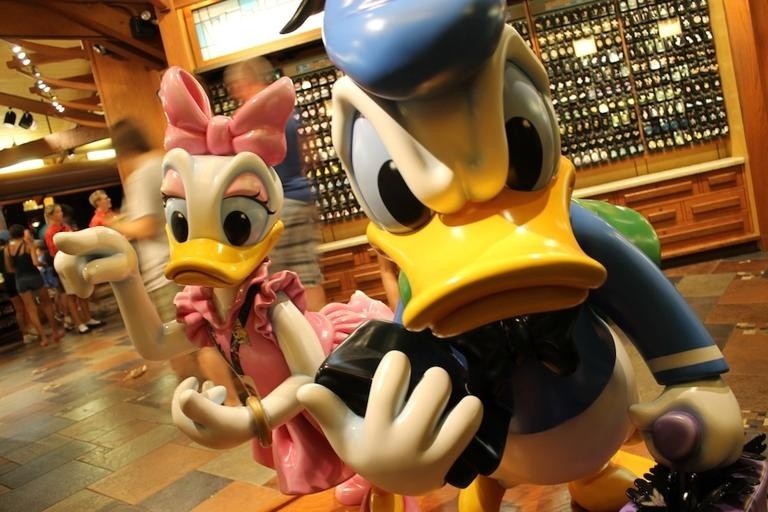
[372,244,408,325]
[0,189,119,346]
[101,118,248,412]
[222,54,326,314]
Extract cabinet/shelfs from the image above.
[156,0,759,315]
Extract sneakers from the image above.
[17,311,75,346]
[84,317,102,326]
[78,323,90,333]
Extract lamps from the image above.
[4,43,69,132]
